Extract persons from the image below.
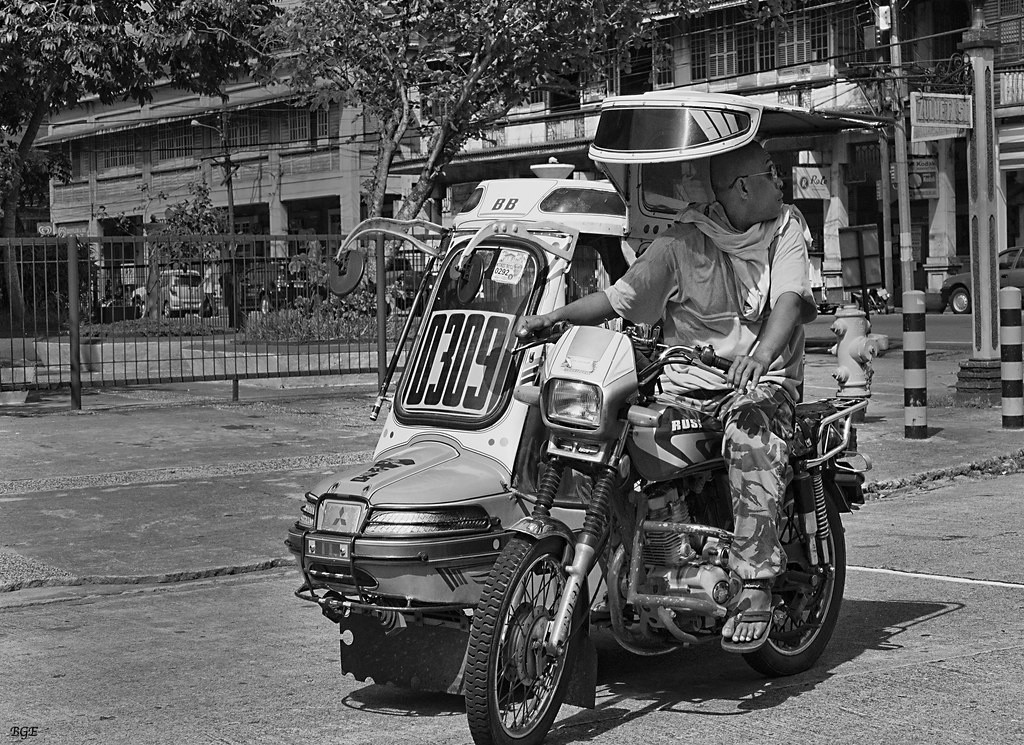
[514,140,817,651]
[193,261,220,319]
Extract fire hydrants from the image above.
[828,304,878,420]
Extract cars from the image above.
[940,246,1024,314]
[130,269,212,319]
[237,264,330,315]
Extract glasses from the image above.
[729,163,778,189]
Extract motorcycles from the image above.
[852,287,890,313]
[465,319,880,744]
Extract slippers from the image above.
[721,604,775,654]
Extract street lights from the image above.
[191,119,242,328]
